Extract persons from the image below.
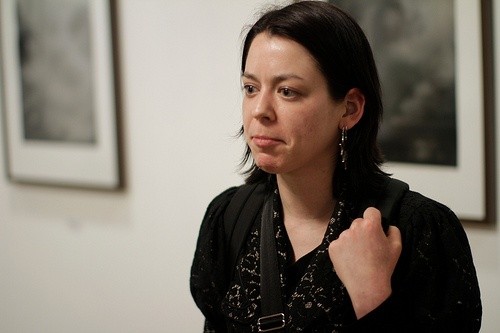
[188,2,485,333]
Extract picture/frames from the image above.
[1,0,123,192]
[296,0,494,223]
